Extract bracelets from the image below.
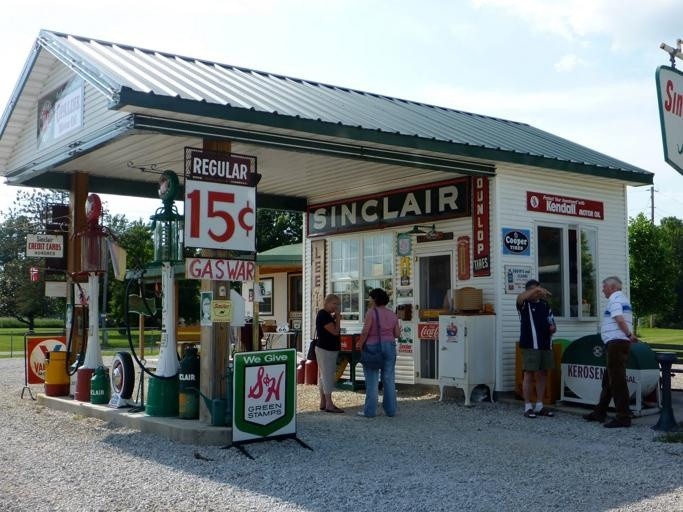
[626,332,631,338]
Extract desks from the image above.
[156,341,200,360]
[262,332,296,349]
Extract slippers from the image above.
[320,402,344,413]
[535,407,555,417]
[524,407,537,419]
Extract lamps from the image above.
[406,224,435,237]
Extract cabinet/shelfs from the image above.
[438,315,496,404]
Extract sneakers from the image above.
[357,410,365,416]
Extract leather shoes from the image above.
[604,417,632,427]
[583,411,606,422]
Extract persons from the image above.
[515,279,556,418]
[579,275,639,428]
[352,287,401,416]
[312,293,345,414]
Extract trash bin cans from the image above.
[44,351,70,396]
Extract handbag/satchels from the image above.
[360,344,385,368]
[307,339,320,360]
